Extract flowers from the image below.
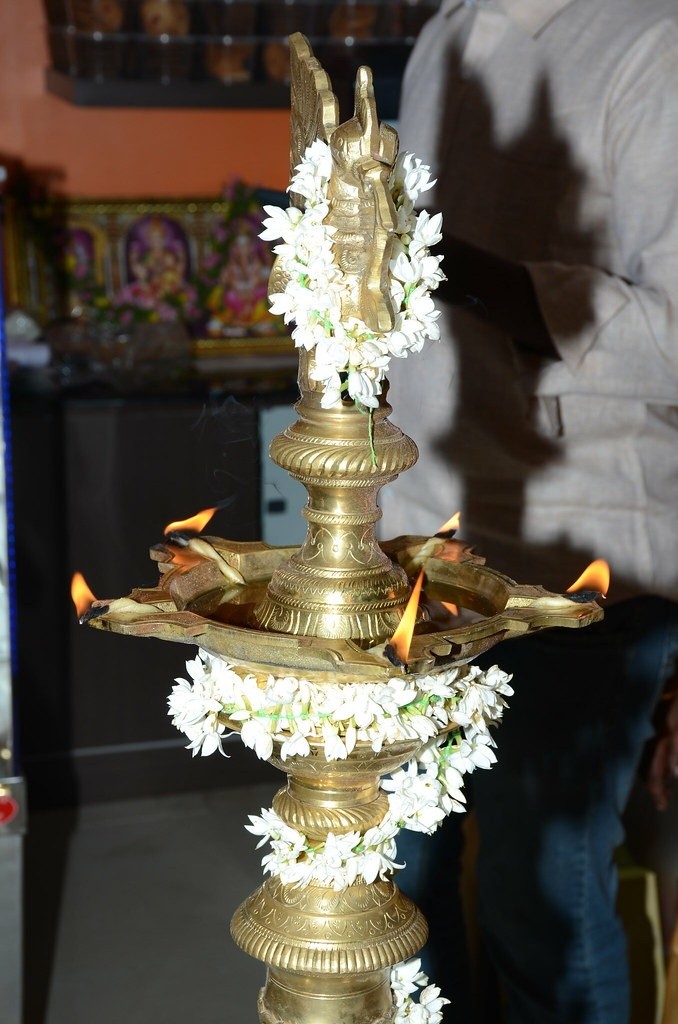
[66,268,203,325]
[257,140,448,472]
[163,645,515,888]
[389,956,449,1024]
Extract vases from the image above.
[66,337,201,379]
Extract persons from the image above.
[400,0,678,1024]
